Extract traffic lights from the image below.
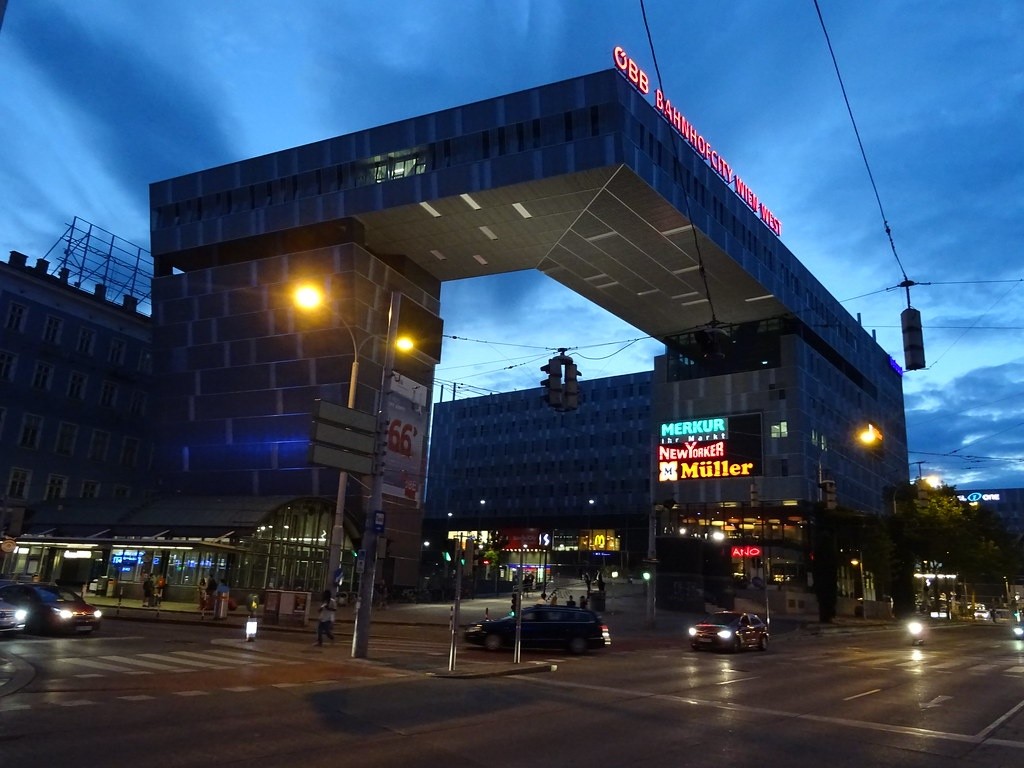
[563,363,583,411]
[641,571,652,580]
[440,549,453,562]
[541,359,562,407]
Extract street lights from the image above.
[291,283,415,599]
[817,427,876,499]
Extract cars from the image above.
[687,611,770,652]
[0,582,102,636]
[973,610,991,620]
[0,597,28,632]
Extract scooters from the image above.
[911,632,925,646]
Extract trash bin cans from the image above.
[106,578,116,597]
[213,590,230,619]
[589,590,605,612]
[33,574,40,583]
[785,586,806,614]
[523,579,532,592]
[855,606,864,618]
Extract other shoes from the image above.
[143,604,148,607]
[328,633,335,641]
[312,641,323,647]
[158,604,161,607]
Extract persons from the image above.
[197,574,229,610]
[511,569,603,611]
[312,590,338,646]
[990,607,997,623]
[139,569,168,607]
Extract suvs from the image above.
[463,604,611,655]
